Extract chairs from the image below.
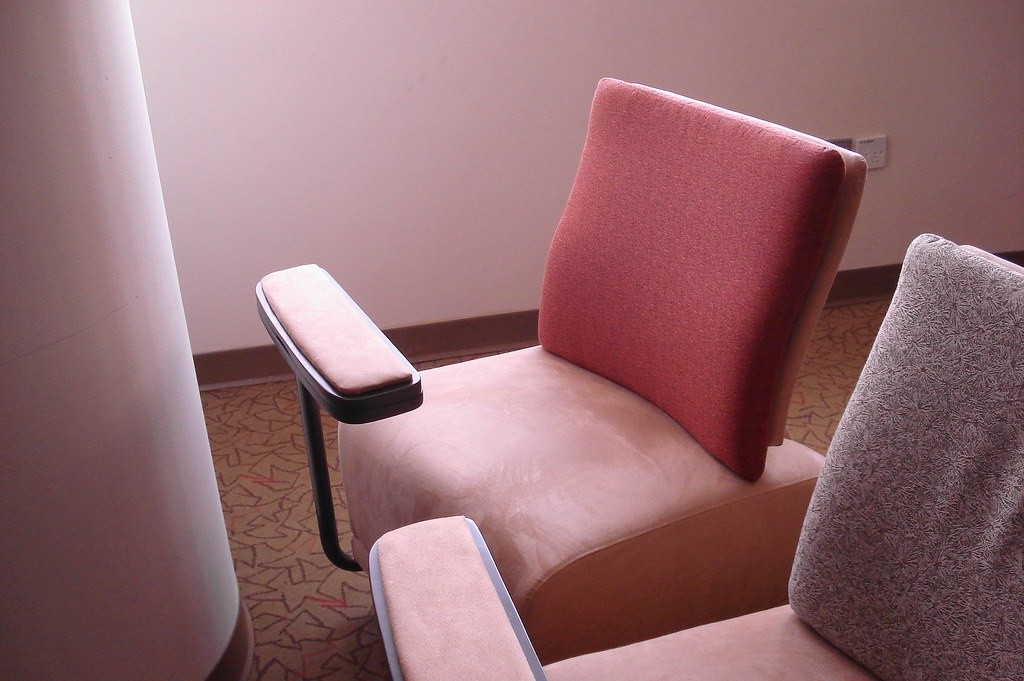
[255,77,1024,681]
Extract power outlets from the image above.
[852,136,887,169]
